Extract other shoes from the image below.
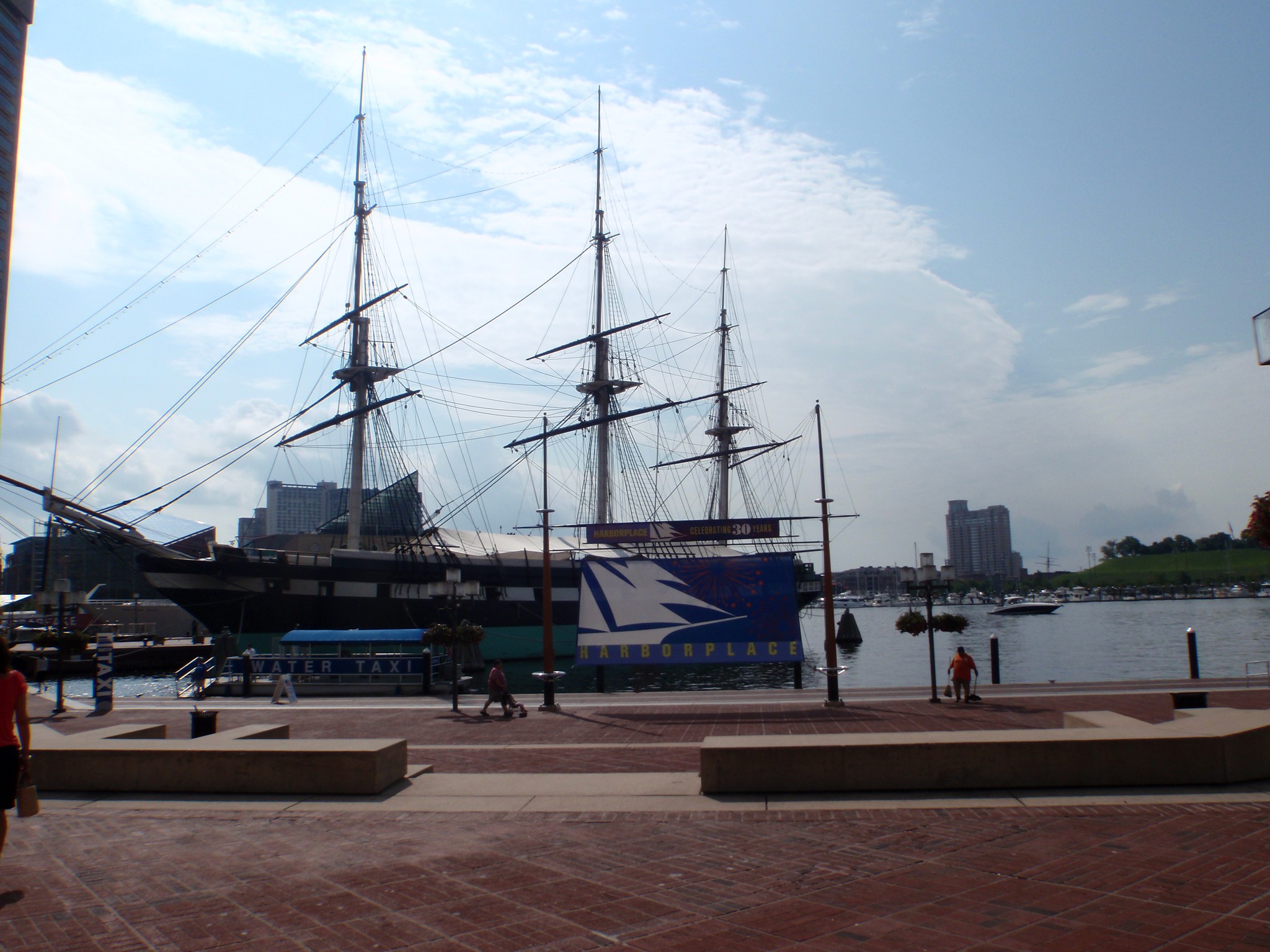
[480,711,489,716]
[504,711,513,715]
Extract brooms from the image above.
[943,656,952,698]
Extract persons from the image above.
[242,644,256,657]
[0,637,30,858]
[480,660,528,716]
[36,652,48,692]
[195,657,207,694]
[947,646,979,703]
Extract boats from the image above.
[986,596,1063,614]
[808,580,1270,609]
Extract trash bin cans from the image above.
[190,711,218,739]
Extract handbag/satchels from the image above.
[16,783,40,819]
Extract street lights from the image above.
[39,576,87,713]
[427,567,482,713]
[133,592,141,634]
[898,552,958,705]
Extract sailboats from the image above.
[1,50,863,679]
